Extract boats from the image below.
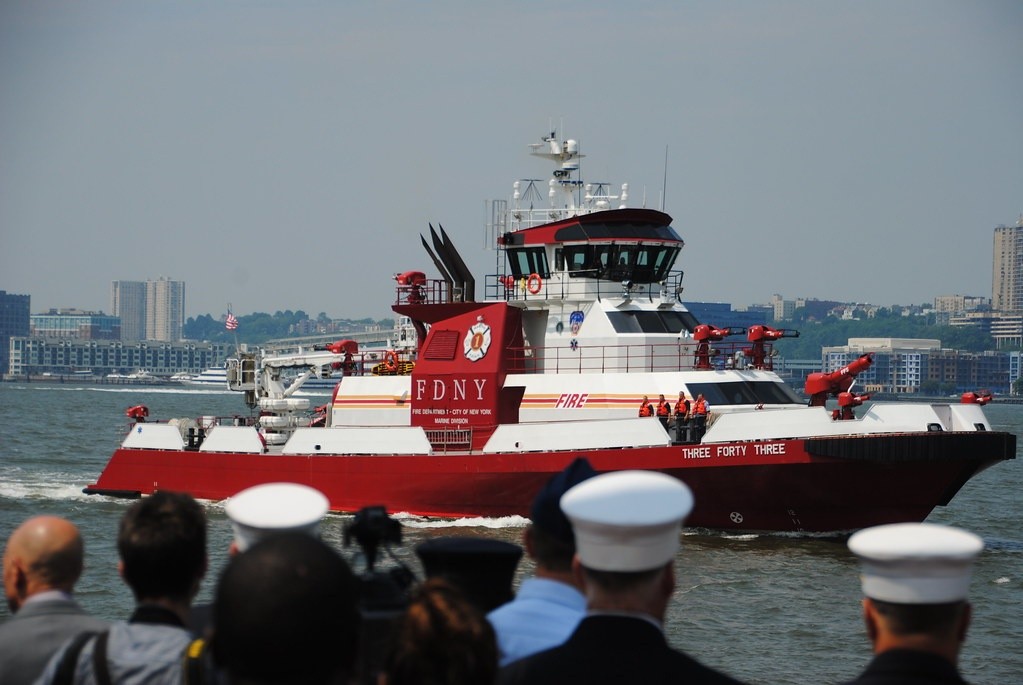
[184,365,230,391]
[83,127,1017,537]
[294,371,342,394]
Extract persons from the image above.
[674,391,690,441]
[656,395,671,434]
[638,396,654,417]
[691,393,710,440]
[0,516,102,685]
[617,257,628,276]
[846,523,982,685]
[36,490,208,685]
[499,470,751,685]
[188,472,587,685]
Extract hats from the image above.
[224,481,328,552]
[559,468,694,571]
[846,522,983,605]
[414,537,523,585]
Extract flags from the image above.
[226,311,239,330]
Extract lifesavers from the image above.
[383,351,398,372]
[527,274,541,296]
